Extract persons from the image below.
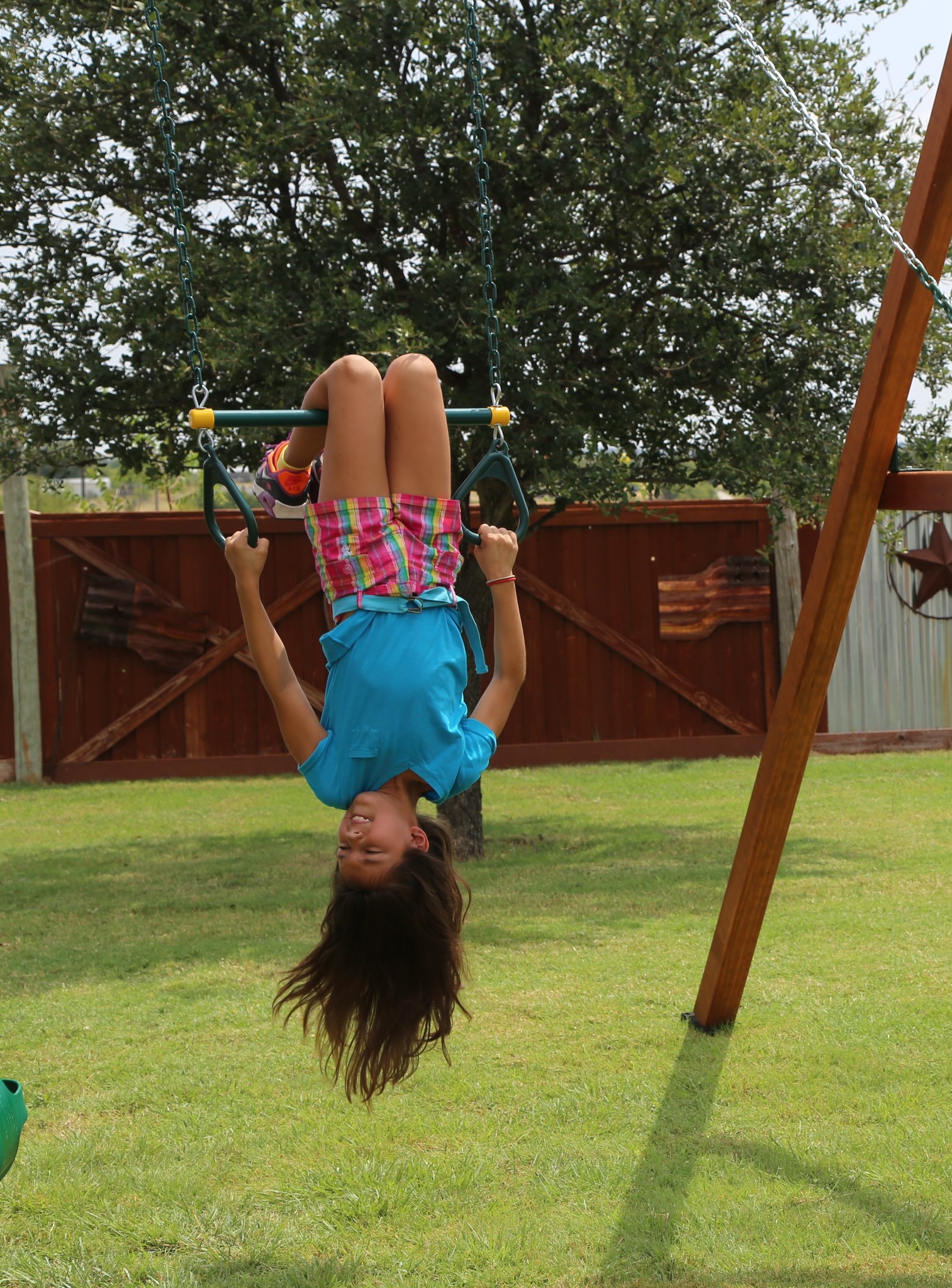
[225,354,527,1105]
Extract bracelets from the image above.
[486,575,516,585]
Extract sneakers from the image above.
[251,439,310,523]
[288,428,324,504]
